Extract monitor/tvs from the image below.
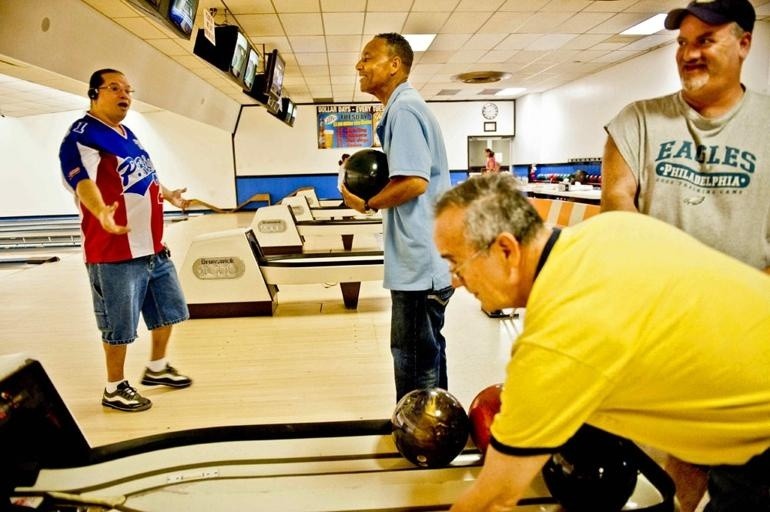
[243,74,283,113]
[267,98,294,124]
[288,104,297,127]
[193,25,249,81]
[233,46,259,93]
[156,0,199,39]
[263,49,285,98]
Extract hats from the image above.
[664,0,755,32]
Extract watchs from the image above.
[364,201,378,215]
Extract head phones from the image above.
[88,89,98,99]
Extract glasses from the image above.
[450,237,496,281]
[98,82,136,93]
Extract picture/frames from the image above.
[484,122,496,131]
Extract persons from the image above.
[434,175,770,512]
[482,148,496,176]
[60,69,190,411]
[602,0,770,274]
[338,154,351,206]
[341,33,455,404]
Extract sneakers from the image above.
[102,362,191,411]
